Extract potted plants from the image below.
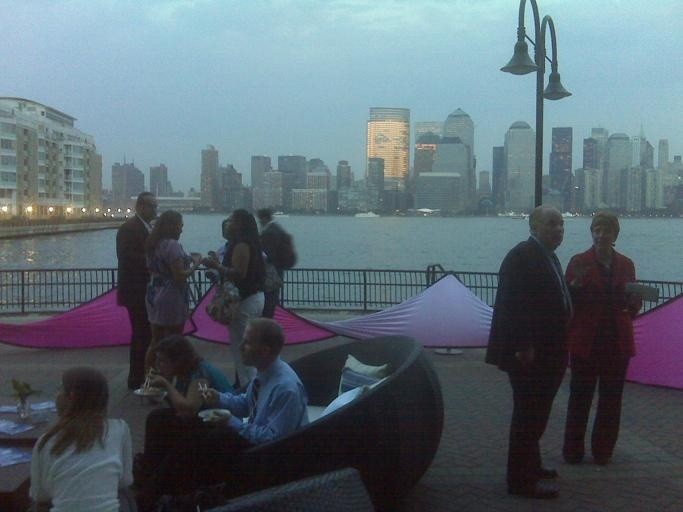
[4,377,45,422]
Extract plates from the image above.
[197,408,231,420]
[133,387,162,397]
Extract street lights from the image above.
[494,0,574,209]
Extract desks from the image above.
[0,394,57,512]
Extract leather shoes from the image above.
[510,480,558,499]
[538,468,557,480]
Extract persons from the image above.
[563,211,641,467]
[26,361,136,512]
[482,204,573,497]
[141,331,243,471]
[113,190,290,391]
[197,316,309,452]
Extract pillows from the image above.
[317,352,389,419]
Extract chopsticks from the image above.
[198,381,209,401]
[142,369,152,390]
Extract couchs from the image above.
[232,333,442,509]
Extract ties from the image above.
[249,378,259,423]
[551,253,571,319]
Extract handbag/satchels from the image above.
[206,280,240,325]
